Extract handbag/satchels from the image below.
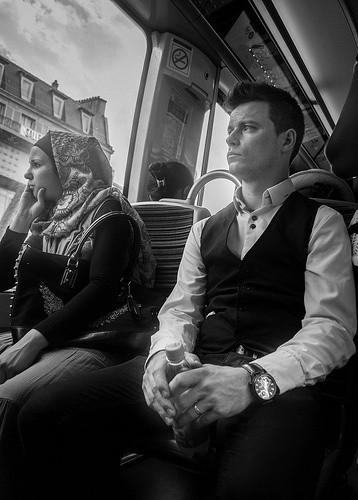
[9,211,142,345]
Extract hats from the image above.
[34,133,53,158]
[148,161,193,198]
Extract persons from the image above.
[146,161,194,202]
[18,82,357,500]
[0,131,156,500]
[324,58,358,277]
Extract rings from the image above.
[192,404,204,417]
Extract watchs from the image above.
[240,363,277,406]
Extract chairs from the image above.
[129,200,211,297]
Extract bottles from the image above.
[165,340,210,459]
